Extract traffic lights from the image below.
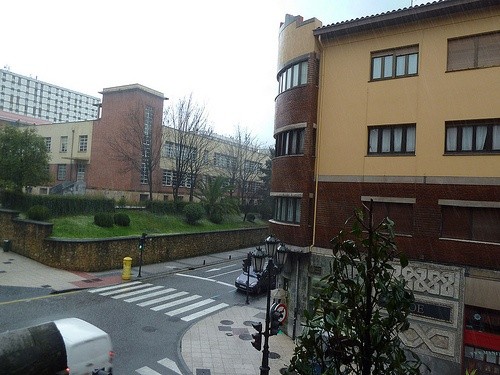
[251,321,262,351]
[138,233,147,250]
[270,310,284,336]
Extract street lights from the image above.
[250,233,289,375]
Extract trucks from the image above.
[0,317,113,375]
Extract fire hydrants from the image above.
[121,255,133,280]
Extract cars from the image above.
[234,261,276,296]
